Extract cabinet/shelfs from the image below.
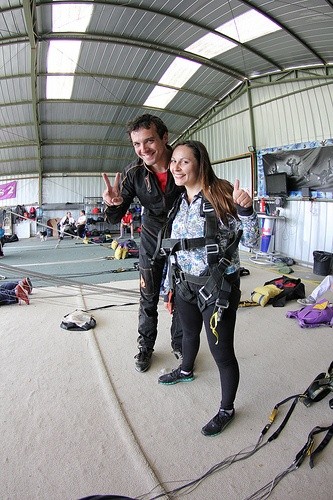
[83,197,104,233]
[1,209,13,236]
[105,220,121,234]
[130,204,140,230]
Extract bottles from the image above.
[260,198,265,212]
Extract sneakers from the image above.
[201,408,235,437]
[158,368,195,385]
[297,297,315,306]
[22,277,32,295]
[171,348,196,370]
[133,348,153,373]
[14,284,30,305]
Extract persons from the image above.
[59,212,75,240]
[101,114,196,373]
[69,210,87,239]
[117,209,135,239]
[158,139,261,437]
[0,277,33,305]
[0,223,5,247]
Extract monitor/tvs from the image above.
[266,172,287,194]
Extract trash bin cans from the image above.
[313,251,333,276]
